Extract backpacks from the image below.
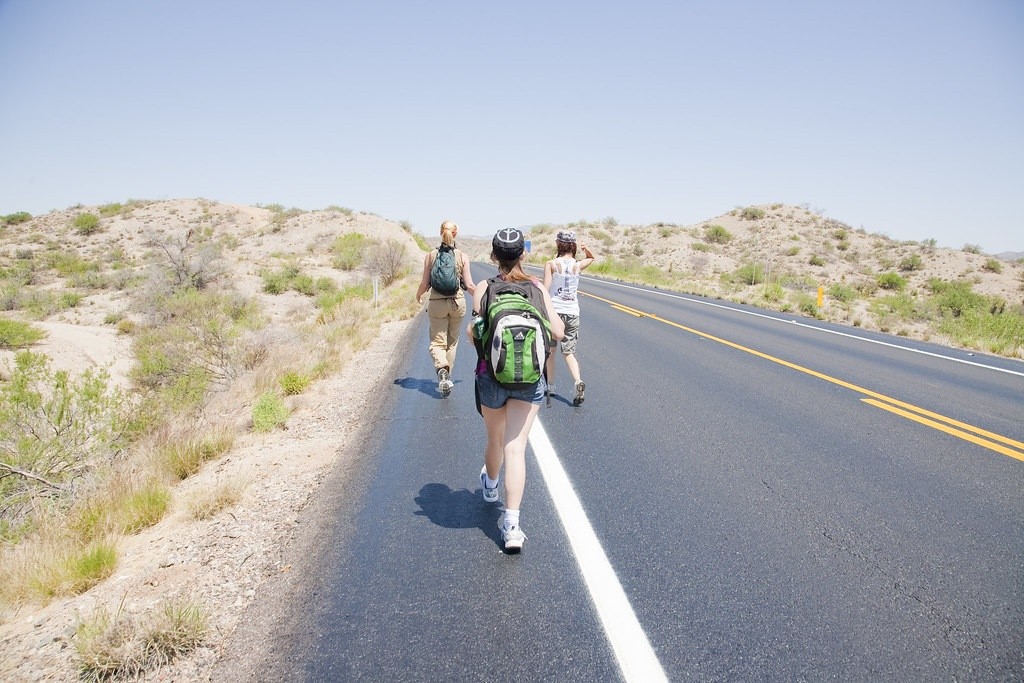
[476,276,552,389]
[429,243,460,295]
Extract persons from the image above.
[466,228,565,549]
[543,230,594,406]
[416,221,476,396]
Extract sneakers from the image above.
[573,380,584,406]
[436,368,453,397]
[497,512,528,551]
[480,463,500,502]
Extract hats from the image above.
[492,228,524,260]
[557,231,576,243]
